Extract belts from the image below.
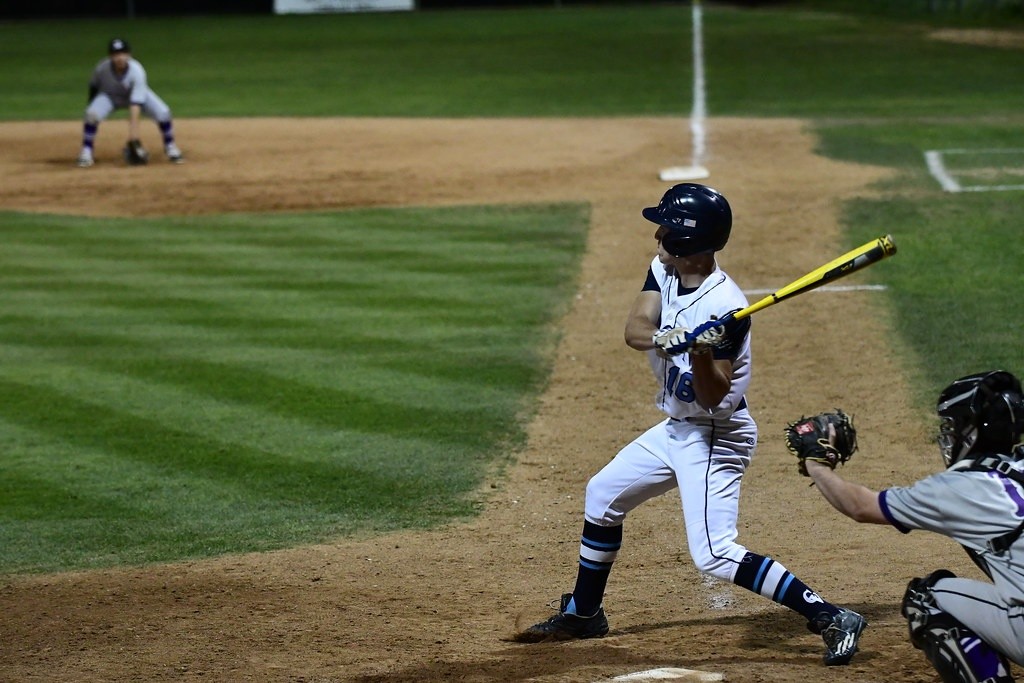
[672,396,747,423]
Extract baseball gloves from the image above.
[785,411,856,478]
[127,139,148,166]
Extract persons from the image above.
[524,182,869,664]
[77,38,186,168]
[785,369,1023,683]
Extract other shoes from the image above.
[163,142,182,158]
[77,145,92,167]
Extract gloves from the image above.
[652,325,695,356]
[688,315,726,355]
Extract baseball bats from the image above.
[660,233,897,355]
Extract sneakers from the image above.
[806,605,869,667]
[514,591,610,643]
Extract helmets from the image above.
[108,38,130,56]
[936,368,1024,471]
[642,183,732,258]
